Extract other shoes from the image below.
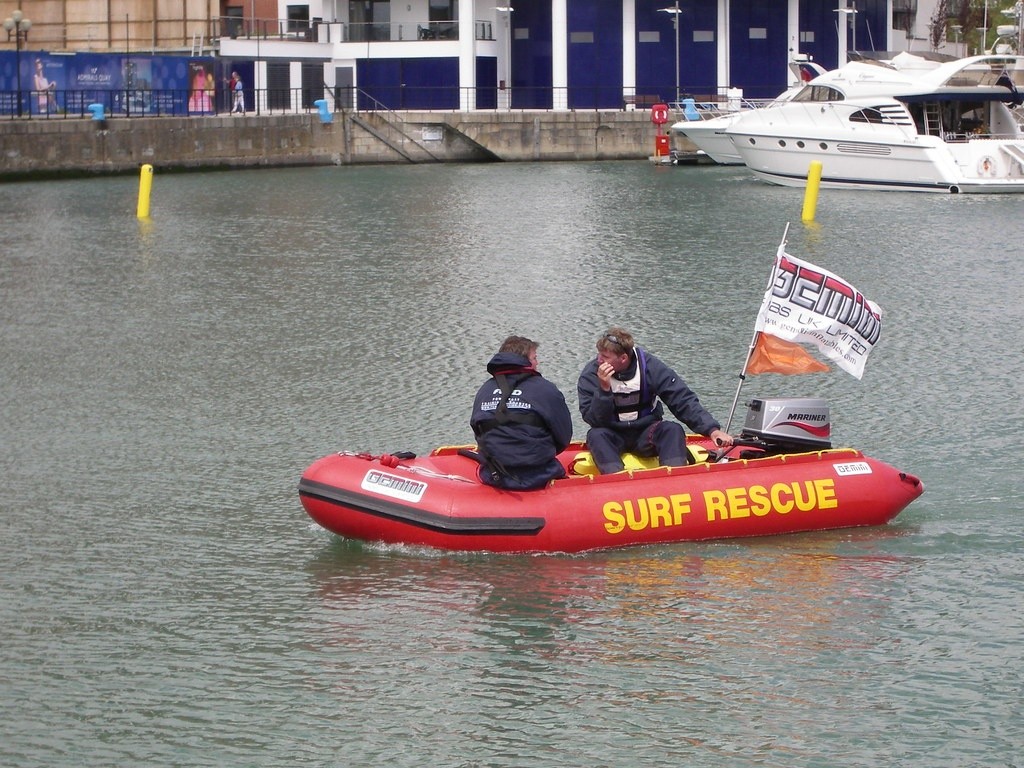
[239,112,244,114]
[231,110,236,113]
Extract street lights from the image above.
[491,0,514,111]
[657,0,683,102]
[4,9,31,116]
[833,1,858,51]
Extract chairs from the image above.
[417,25,457,40]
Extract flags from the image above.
[755,246,886,382]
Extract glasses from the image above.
[603,332,628,355]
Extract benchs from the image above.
[623,94,728,109]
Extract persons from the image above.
[33,59,61,115]
[470,333,574,492]
[577,328,733,476]
[222,71,247,116]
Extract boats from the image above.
[667,0,1024,194]
[297,227,926,553]
